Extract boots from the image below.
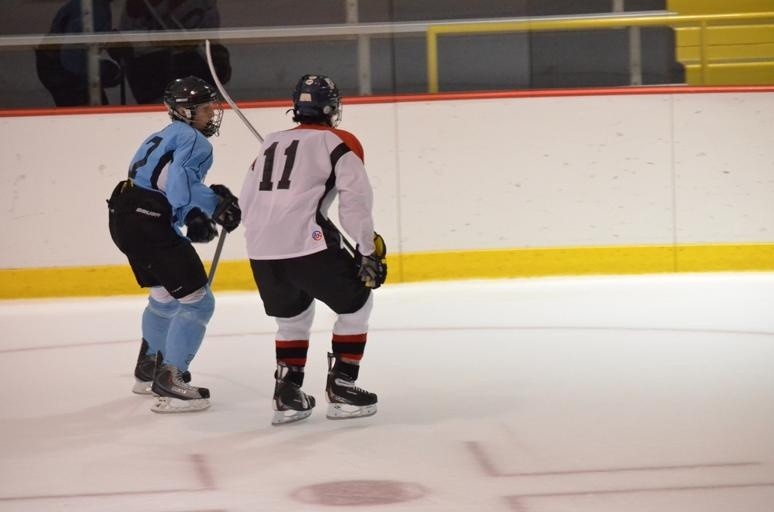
[135,336,190,384]
[273,360,315,411]
[326,351,377,405]
[152,349,210,400]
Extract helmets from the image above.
[163,75,223,138]
[293,74,342,128]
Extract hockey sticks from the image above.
[205,40,356,257]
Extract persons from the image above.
[117,1,232,103]
[41,1,120,109]
[236,72,386,411]
[105,74,242,400]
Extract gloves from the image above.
[210,184,241,233]
[355,232,387,289]
[185,206,217,243]
